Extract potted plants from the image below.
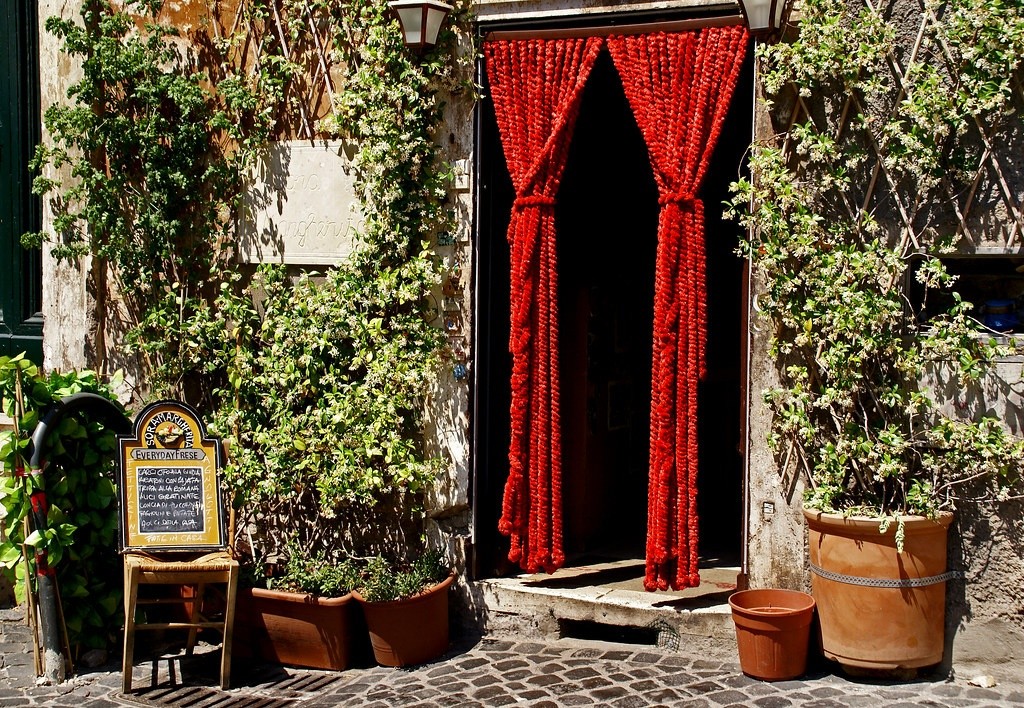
[239,532,352,671]
[718,0,1024,682]
[350,546,457,668]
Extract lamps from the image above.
[737,0,788,44]
[387,0,455,56]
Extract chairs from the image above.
[121,439,240,695]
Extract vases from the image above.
[179,540,268,657]
[727,589,815,682]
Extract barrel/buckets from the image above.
[730,588,815,679]
